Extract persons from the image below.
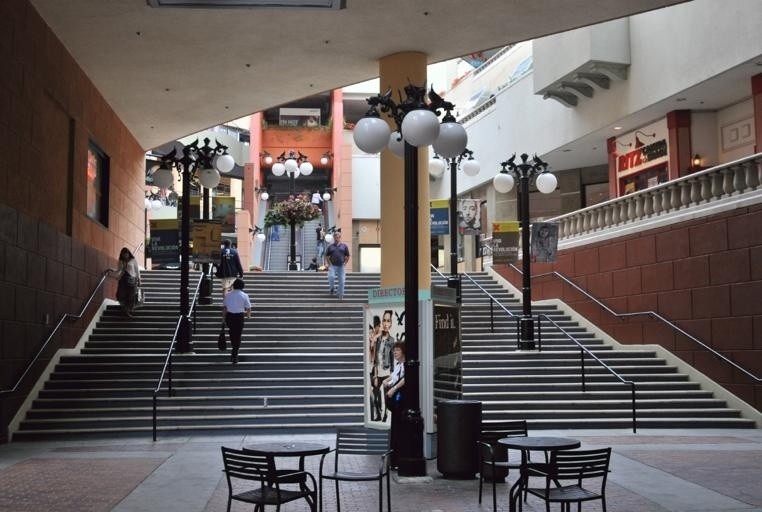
[367,309,404,423]
[322,231,350,301]
[315,223,324,250]
[219,278,252,365]
[534,226,556,260]
[104,245,143,321]
[456,197,486,233]
[304,255,321,273]
[215,239,244,297]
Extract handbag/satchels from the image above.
[136,287,144,305]
[218,331,227,350]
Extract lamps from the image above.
[320,151,335,167]
[258,149,273,165]
[248,223,266,244]
[253,185,270,203]
[689,152,703,171]
[322,185,338,202]
[608,138,632,161]
[632,130,656,150]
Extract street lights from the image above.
[271,148,315,272]
[492,152,557,351]
[180,136,235,306]
[152,145,221,354]
[428,147,481,306]
[351,76,468,477]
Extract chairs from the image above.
[475,418,535,511]
[516,446,612,512]
[316,427,396,512]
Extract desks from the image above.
[219,446,319,512]
[496,436,582,510]
[241,442,331,512]
